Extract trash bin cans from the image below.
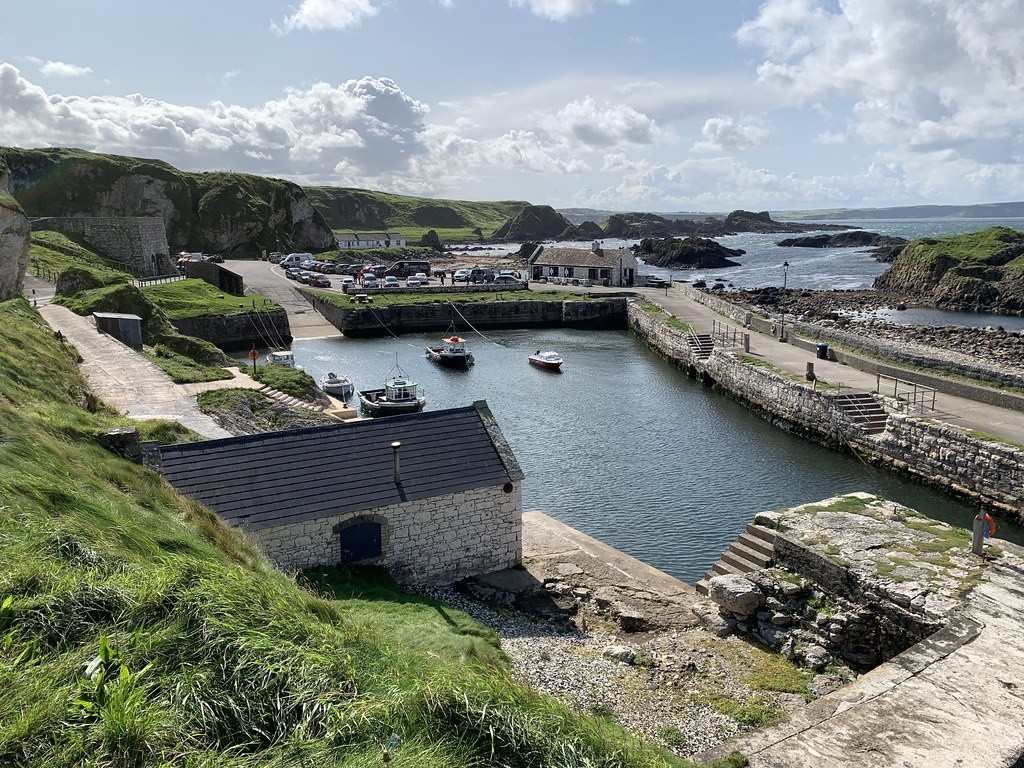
[816,342,828,358]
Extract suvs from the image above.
[471,267,495,282]
[494,276,524,283]
[454,269,472,281]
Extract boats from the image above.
[356,352,426,414]
[426,336,471,363]
[267,351,295,367]
[321,372,354,396]
[528,350,563,368]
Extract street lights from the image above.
[777,260,789,343]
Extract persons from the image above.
[357,271,361,284]
[451,274,455,286]
[312,298,316,310]
[472,274,476,285]
[353,272,357,283]
[466,274,470,285]
[440,274,444,285]
[56,329,63,343]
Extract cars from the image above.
[285,267,331,287]
[406,273,428,286]
[269,252,287,263]
[176,251,223,269]
[300,260,399,290]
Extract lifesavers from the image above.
[248,350,259,360]
[394,379,406,385]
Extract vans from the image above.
[384,261,431,277]
[279,253,313,269]
